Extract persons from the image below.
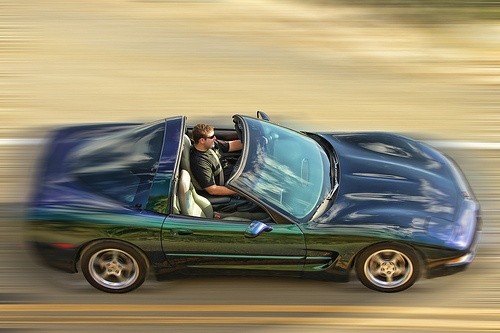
[189,124,265,213]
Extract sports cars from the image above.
[25,111,482,295]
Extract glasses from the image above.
[201,134,215,139]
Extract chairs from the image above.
[178,134,218,192]
[178,170,255,223]
